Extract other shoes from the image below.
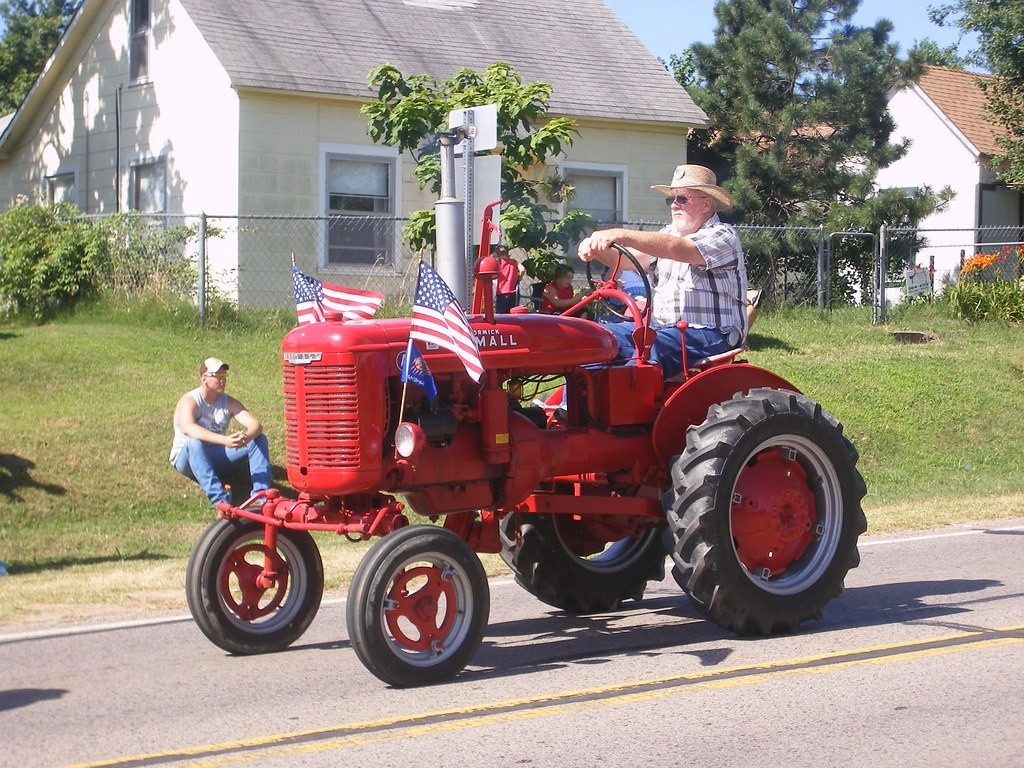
[552,408,568,421]
[212,506,222,522]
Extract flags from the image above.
[528,381,568,428]
[401,340,437,400]
[291,267,383,329]
[409,259,485,383]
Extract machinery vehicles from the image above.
[183,124,867,688]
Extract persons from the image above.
[576,165,748,380]
[539,263,594,322]
[168,358,271,518]
[472,243,525,314]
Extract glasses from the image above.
[204,372,228,380]
[666,195,707,206]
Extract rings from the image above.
[577,249,581,252]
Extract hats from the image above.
[200,358,229,374]
[651,165,733,211]
